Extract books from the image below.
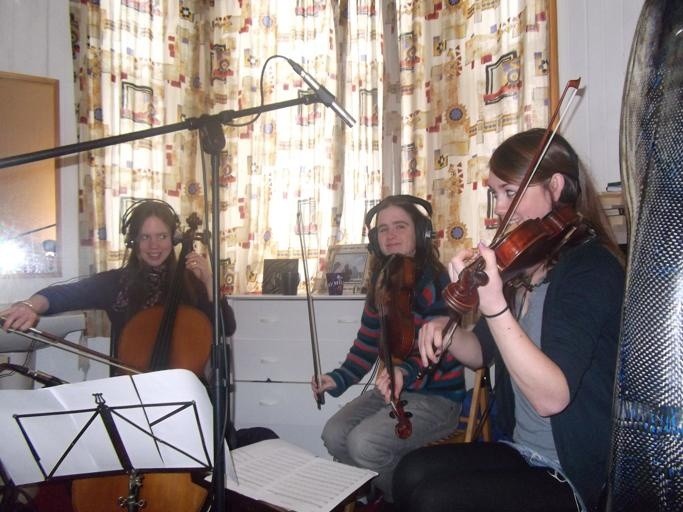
[208,437,379,512]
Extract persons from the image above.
[390,127,626,512]
[1,202,237,378]
[311,196,465,502]
[334,262,362,283]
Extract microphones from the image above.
[286,58,357,128]
[10,364,68,387]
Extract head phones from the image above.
[121,198,183,249]
[364,195,437,258]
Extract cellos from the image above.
[70,212,219,511]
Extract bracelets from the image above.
[20,300,41,314]
[483,302,510,320]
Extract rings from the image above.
[194,261,197,265]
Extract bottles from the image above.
[221,259,234,295]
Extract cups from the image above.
[326,272,344,296]
[282,271,298,295]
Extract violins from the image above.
[441,204,585,313]
[374,253,414,440]
